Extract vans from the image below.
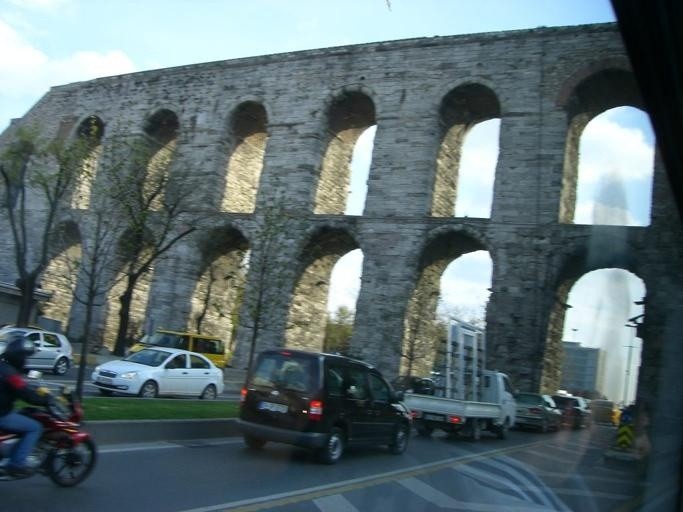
[234,348,412,465]
[124,330,227,372]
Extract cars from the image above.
[91,346,226,399]
[0,327,74,376]
[390,375,434,395]
[514,391,624,433]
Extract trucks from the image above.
[402,369,520,442]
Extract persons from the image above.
[208,342,216,353]
[160,337,170,346]
[0,336,55,478]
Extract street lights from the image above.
[623,346,636,405]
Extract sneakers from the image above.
[10,466,34,476]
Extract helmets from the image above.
[5,339,35,360]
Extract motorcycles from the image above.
[0,386,98,487]
